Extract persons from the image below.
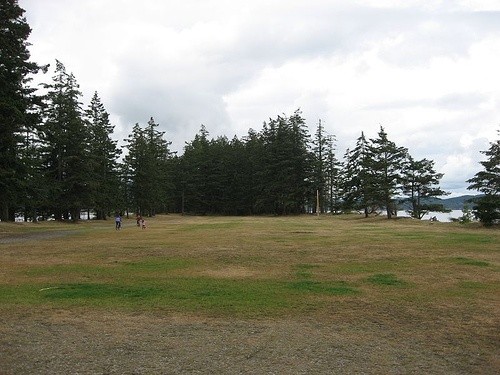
[114,214,122,231]
[136,215,142,228]
[141,218,146,229]
